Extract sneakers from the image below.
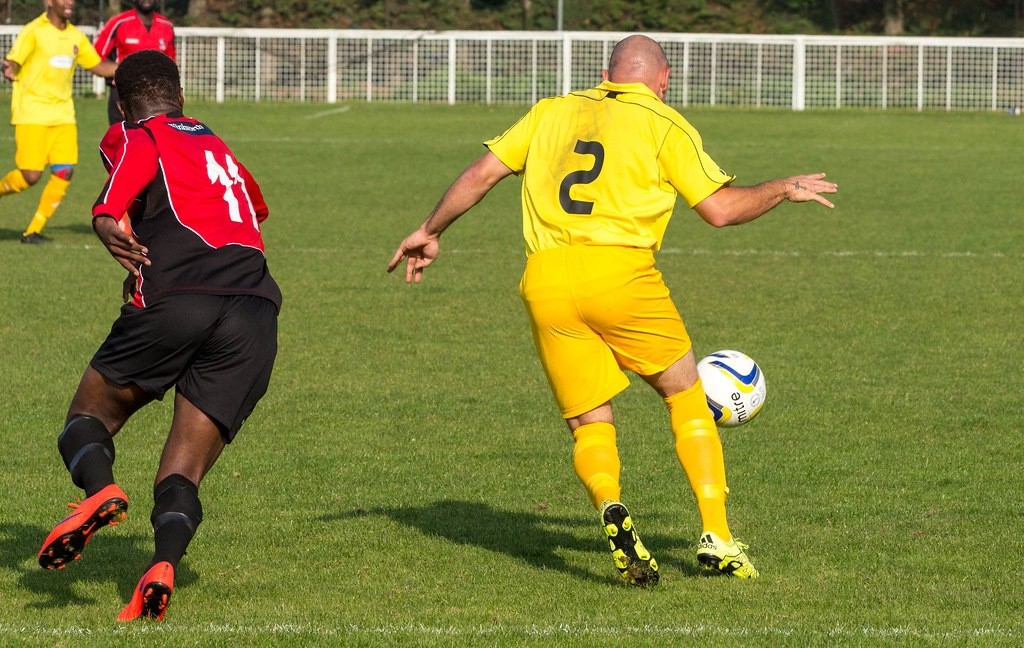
[600,500,661,591]
[698,531,758,580]
[117,562,173,623]
[36,481,131,570]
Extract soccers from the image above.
[697,349,767,429]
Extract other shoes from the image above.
[20,231,55,246]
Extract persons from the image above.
[386,36,838,592]
[93,0,177,236]
[0,0,119,244]
[38,49,283,623]
